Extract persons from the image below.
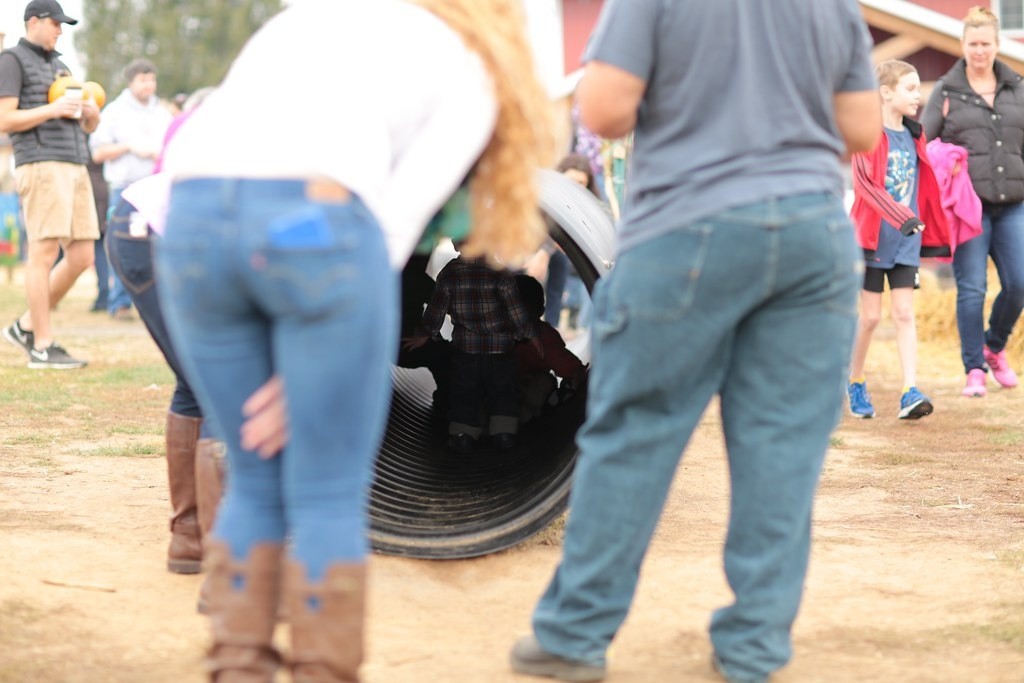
[848,58,968,419]
[392,232,590,452]
[148,1,560,683]
[0,0,102,372]
[918,6,1024,398]
[523,153,599,335]
[89,59,239,575]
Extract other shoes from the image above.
[489,416,519,449]
[92,298,108,310]
[114,304,134,320]
[448,421,482,454]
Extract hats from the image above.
[24,0,77,25]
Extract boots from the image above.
[168,409,224,574]
[199,539,368,683]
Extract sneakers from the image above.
[3,320,34,354]
[964,369,987,397]
[983,344,1018,388]
[511,634,607,683]
[898,387,933,419]
[846,376,875,418]
[28,342,87,370]
[711,652,730,683]
